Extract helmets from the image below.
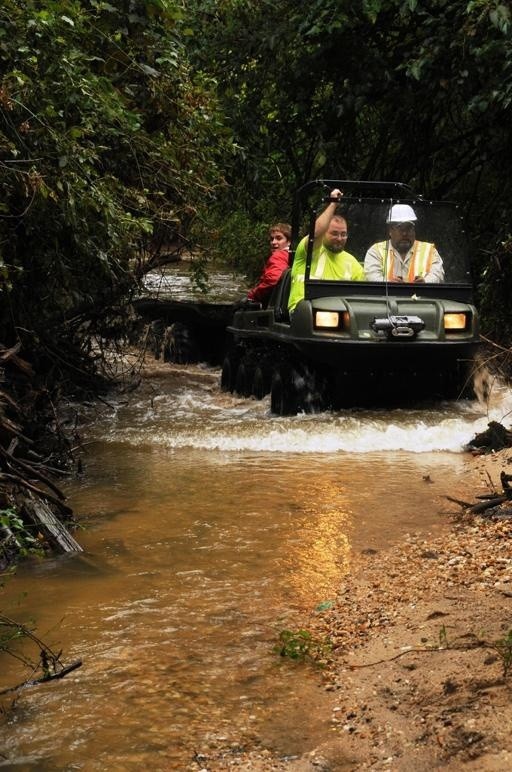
[387,203,417,224]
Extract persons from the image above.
[288,189,366,328]
[247,223,293,301]
[364,203,445,284]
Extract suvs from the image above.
[220,178,480,418]
